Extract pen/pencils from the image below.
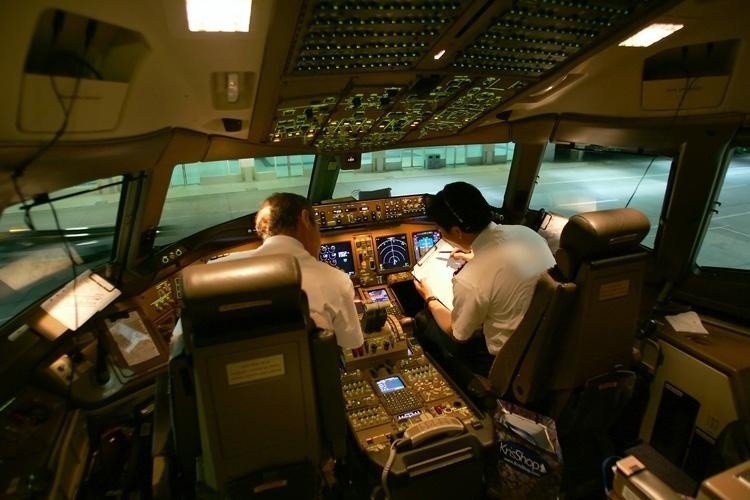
[439,250,453,254]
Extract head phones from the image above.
[435,190,472,233]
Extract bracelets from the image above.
[424,296,439,309]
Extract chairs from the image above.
[168,253,348,492]
[474,208,658,439]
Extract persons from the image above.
[168,192,364,359]
[413,182,557,378]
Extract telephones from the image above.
[403,416,464,447]
